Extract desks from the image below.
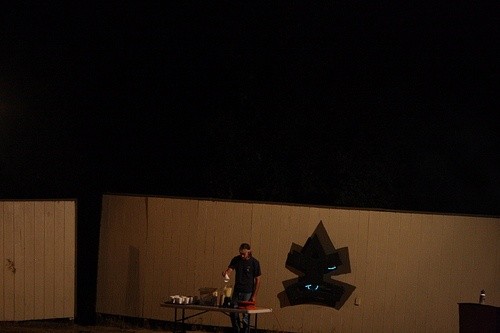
[160,298,273,333]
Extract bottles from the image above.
[479,288,485,304]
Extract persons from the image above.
[225,243,261,332]
